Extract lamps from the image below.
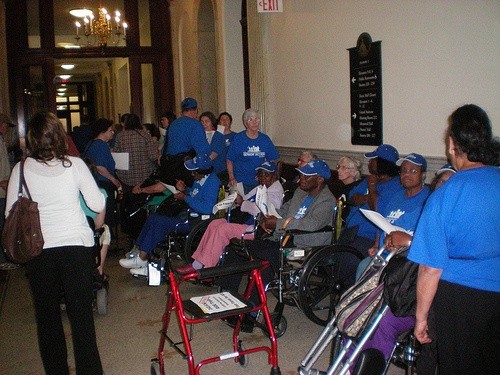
[74,0,130,56]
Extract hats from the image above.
[254,162,277,173]
[181,98,198,110]
[184,155,213,169]
[363,144,399,162]
[294,159,331,181]
[437,165,456,174]
[395,154,428,170]
[0,112,16,127]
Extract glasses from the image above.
[336,164,357,171]
[399,169,420,176]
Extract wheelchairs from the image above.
[86,215,111,315]
[130,184,226,287]
[220,201,366,338]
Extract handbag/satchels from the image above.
[0,157,44,264]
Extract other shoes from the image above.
[222,316,255,334]
[175,264,196,275]
[118,253,149,276]
[100,273,110,281]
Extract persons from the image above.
[163,98,211,190]
[354,152,433,286]
[407,104,500,375]
[0,109,109,375]
[225,106,283,202]
[0,98,500,375]
